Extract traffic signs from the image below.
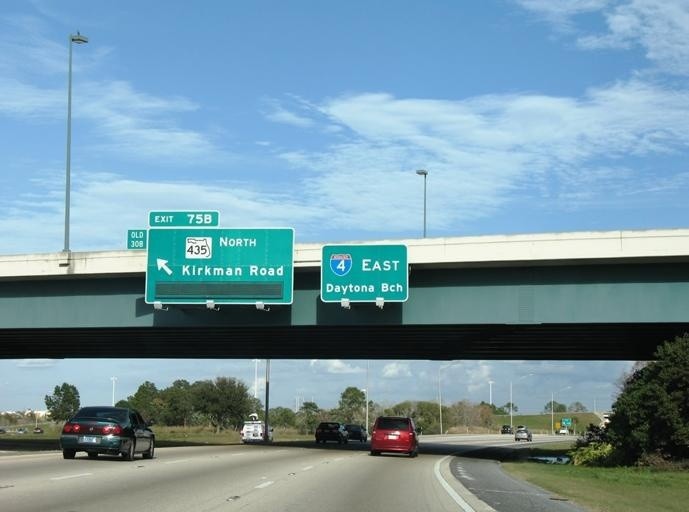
[144,226,293,306]
[148,209,222,229]
[561,418,572,427]
[320,243,411,305]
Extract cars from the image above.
[33,427,44,434]
[60,404,158,459]
[15,427,28,434]
[555,428,574,435]
[0,427,6,434]
[368,415,422,457]
[500,424,535,444]
[344,423,369,441]
[315,420,350,444]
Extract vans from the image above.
[239,420,276,444]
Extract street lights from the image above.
[509,372,535,428]
[487,380,496,404]
[63,30,95,252]
[551,385,573,434]
[252,358,261,401]
[414,167,429,237]
[110,375,117,407]
